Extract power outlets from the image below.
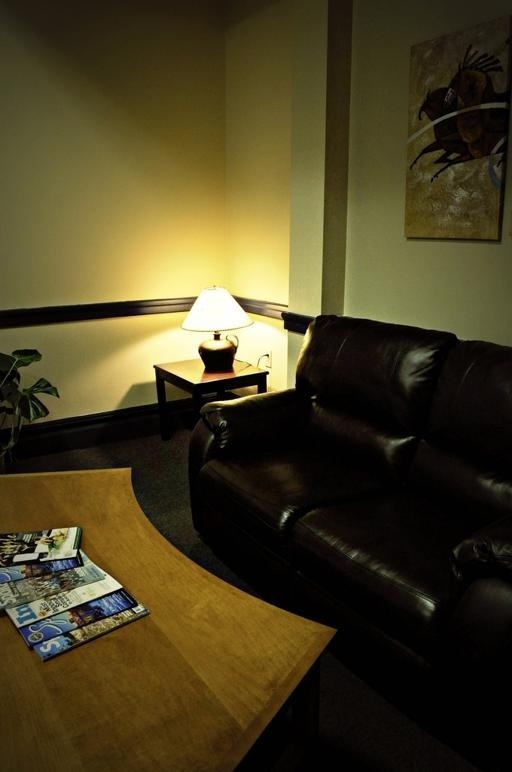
[264,351,272,368]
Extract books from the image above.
[0,526,152,663]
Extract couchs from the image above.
[188,313,511,772]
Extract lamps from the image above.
[180,284,254,373]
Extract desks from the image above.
[0,463,340,771]
[152,359,268,441]
